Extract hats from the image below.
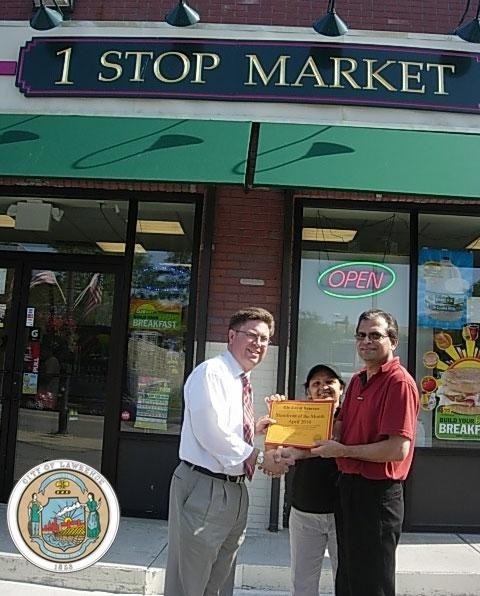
[306,363,343,384]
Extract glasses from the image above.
[232,327,271,346]
[352,331,390,341]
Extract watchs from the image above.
[256,450,264,465]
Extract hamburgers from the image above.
[441,368,480,415]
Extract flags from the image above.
[30,269,56,292]
[80,273,104,322]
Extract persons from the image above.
[265,362,343,596]
[45,351,59,384]
[163,305,297,596]
[258,309,421,595]
[83,492,103,544]
[27,493,43,536]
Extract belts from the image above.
[181,459,246,485]
